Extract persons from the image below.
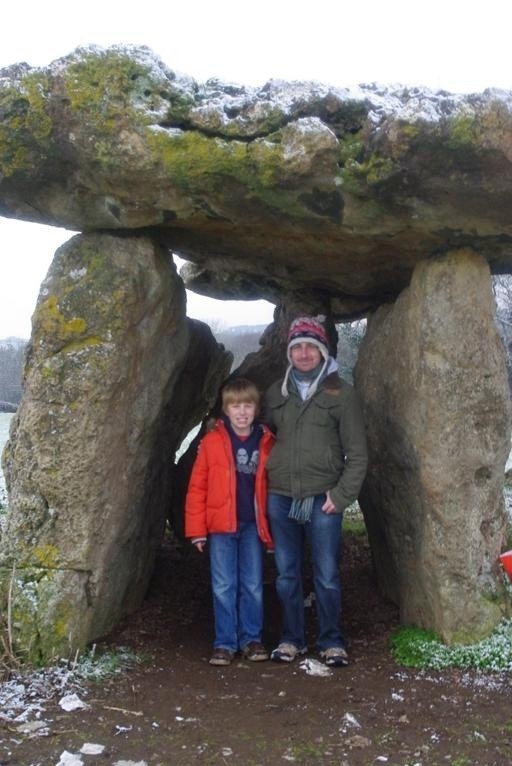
[195,313,370,668]
[184,376,277,666]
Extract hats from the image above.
[286,316,330,367]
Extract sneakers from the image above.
[244,641,269,661]
[270,642,309,663]
[318,647,352,668]
[208,648,235,666]
